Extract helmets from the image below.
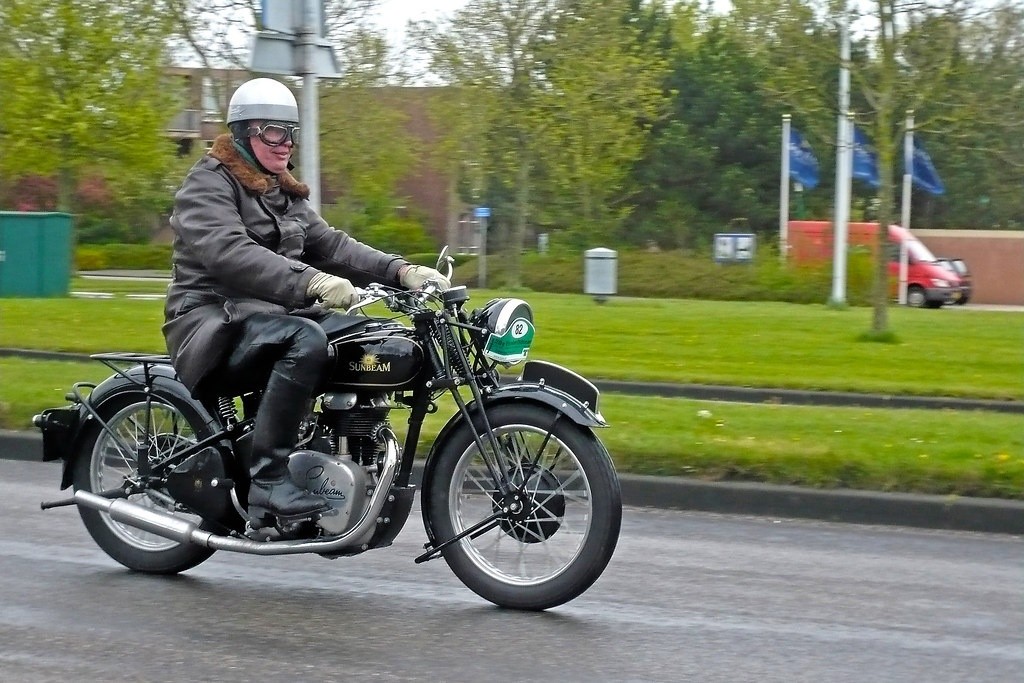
[227,78,299,146]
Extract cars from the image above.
[933,258,971,304]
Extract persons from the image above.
[162,78,451,520]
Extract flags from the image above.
[852,125,883,187]
[789,127,820,190]
[911,133,945,195]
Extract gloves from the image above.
[306,271,360,317]
[399,265,451,291]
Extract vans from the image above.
[780,221,962,307]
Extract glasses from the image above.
[248,120,300,147]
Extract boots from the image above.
[248,370,332,518]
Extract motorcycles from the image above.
[30,248,622,609]
